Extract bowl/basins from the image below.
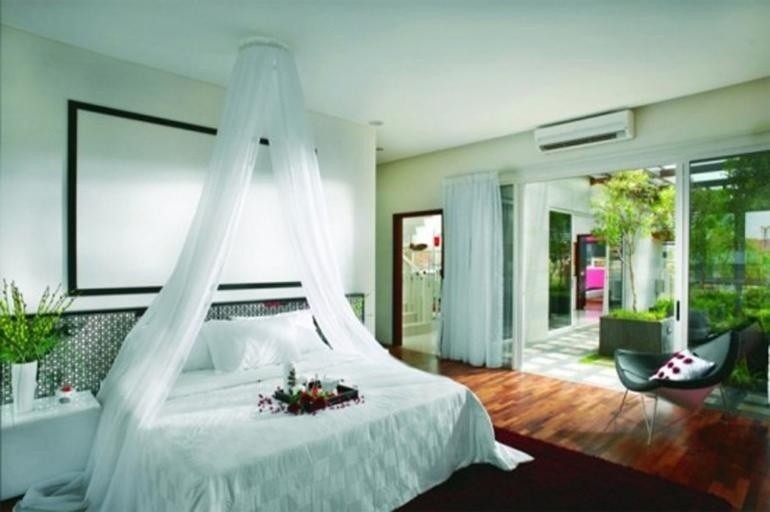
[55,386,74,398]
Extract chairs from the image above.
[614,330,740,447]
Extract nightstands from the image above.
[2,390,103,503]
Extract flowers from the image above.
[255,369,366,416]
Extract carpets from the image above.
[396,424,735,512]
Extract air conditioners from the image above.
[535,108,633,153]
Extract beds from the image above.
[104,346,474,510]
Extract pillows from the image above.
[181,308,329,373]
[649,346,715,382]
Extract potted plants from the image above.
[588,168,676,357]
[0,278,86,415]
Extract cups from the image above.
[320,377,338,392]
